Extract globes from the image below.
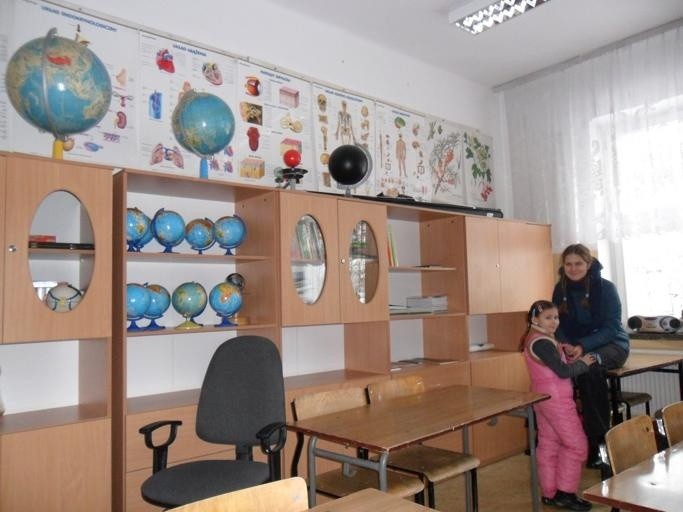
[125,206,248,255]
[173,90,236,178]
[4,27,114,159]
[127,283,243,332]
[329,141,372,197]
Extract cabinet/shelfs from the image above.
[464,215,554,316]
[1,150,115,511]
[277,190,390,328]
[385,202,475,481]
[469,350,535,469]
[113,168,279,511]
[281,373,392,506]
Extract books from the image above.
[390,356,457,372]
[293,220,448,315]
[29,240,94,250]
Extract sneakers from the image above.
[540,497,555,505]
[555,490,593,511]
[587,442,611,469]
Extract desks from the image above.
[582,353,683,511]
[285,384,552,510]
[299,486,443,511]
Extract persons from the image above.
[519,300,596,512]
[524,242,631,472]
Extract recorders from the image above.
[627,314,681,337]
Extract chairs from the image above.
[137,335,288,509]
[164,475,311,510]
[364,376,481,509]
[289,384,426,508]
